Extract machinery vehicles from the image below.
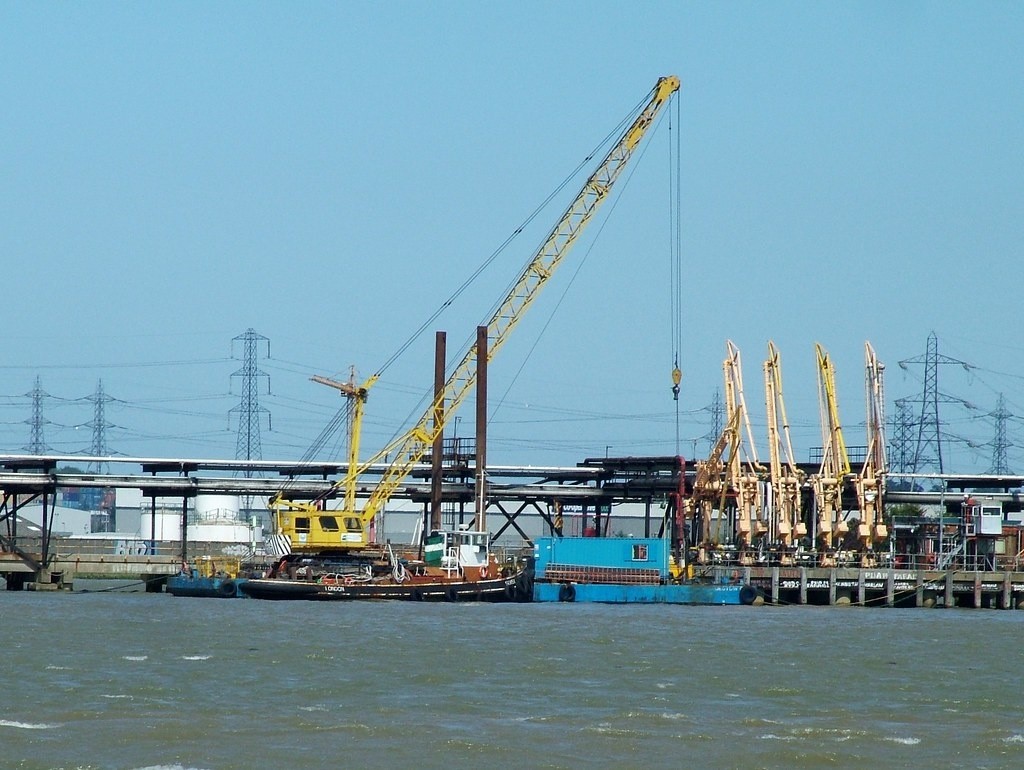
[270,77,682,572]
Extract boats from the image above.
[166,556,249,598]
[239,455,527,602]
[517,493,765,604]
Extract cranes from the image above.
[671,340,893,568]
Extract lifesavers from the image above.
[505,584,516,600]
[526,579,533,592]
[344,577,353,586]
[516,578,530,594]
[181,562,187,572]
[411,587,424,601]
[445,587,459,602]
[220,581,237,597]
[740,585,757,604]
[559,585,576,602]
[478,565,488,578]
